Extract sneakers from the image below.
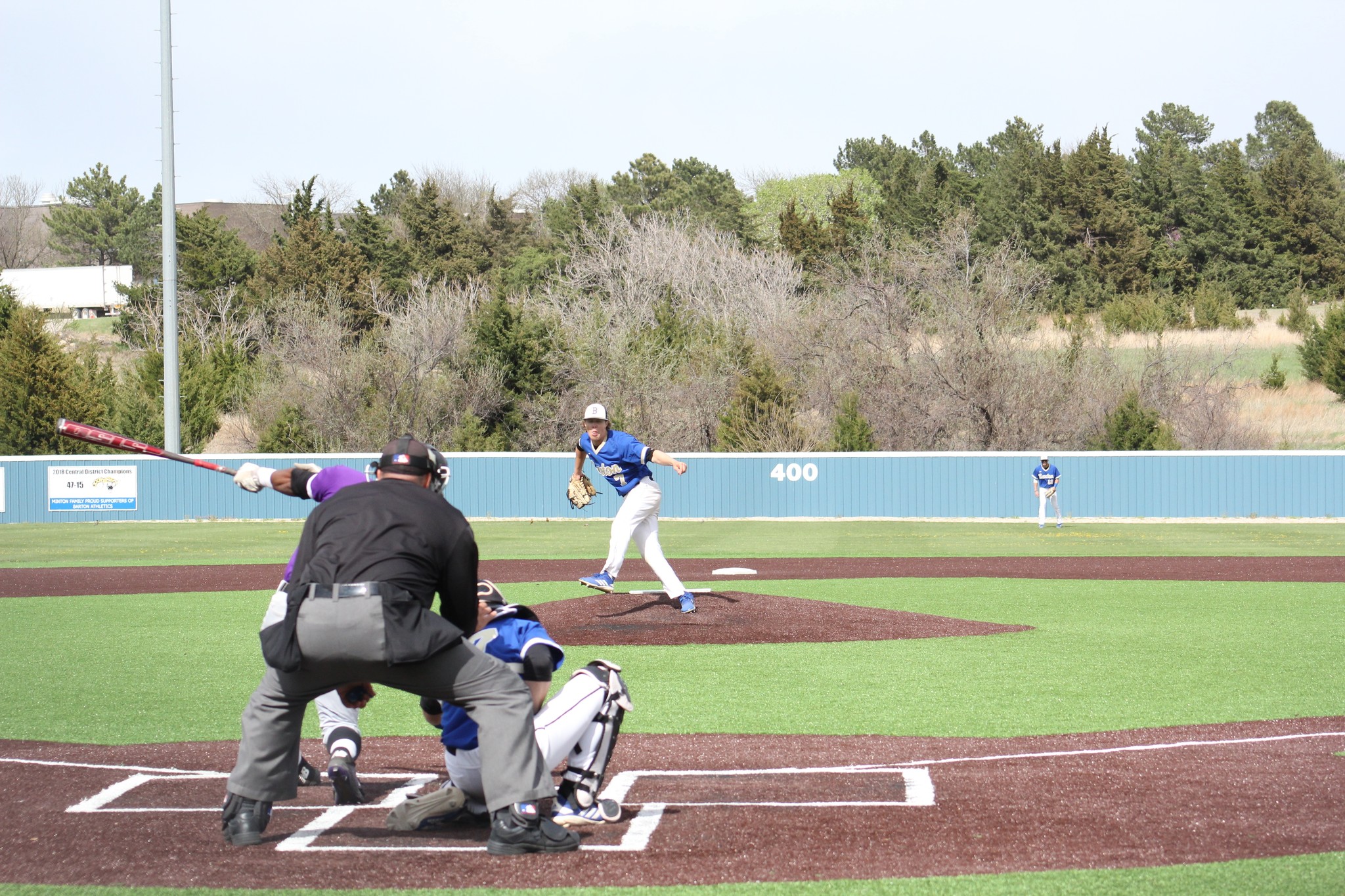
[550,795,606,826]
[578,573,614,593]
[1039,524,1043,528]
[295,759,321,786]
[386,787,464,831]
[329,749,364,805]
[220,790,272,845]
[488,810,580,854]
[1056,523,1062,528]
[679,591,695,612]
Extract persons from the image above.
[233,443,451,805]
[386,579,633,832]
[220,433,581,854]
[1031,456,1064,528]
[568,404,696,613]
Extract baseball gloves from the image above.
[567,473,596,508]
[1044,489,1055,498]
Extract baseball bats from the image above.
[56,418,237,476]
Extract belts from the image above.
[305,582,401,598]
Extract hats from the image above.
[380,434,429,475]
[477,579,504,604]
[583,403,609,420]
[1040,455,1047,460]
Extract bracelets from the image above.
[1035,491,1037,492]
[1054,483,1057,487]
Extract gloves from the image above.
[233,462,263,492]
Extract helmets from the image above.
[421,443,449,494]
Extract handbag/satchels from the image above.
[384,593,465,665]
[260,586,305,671]
